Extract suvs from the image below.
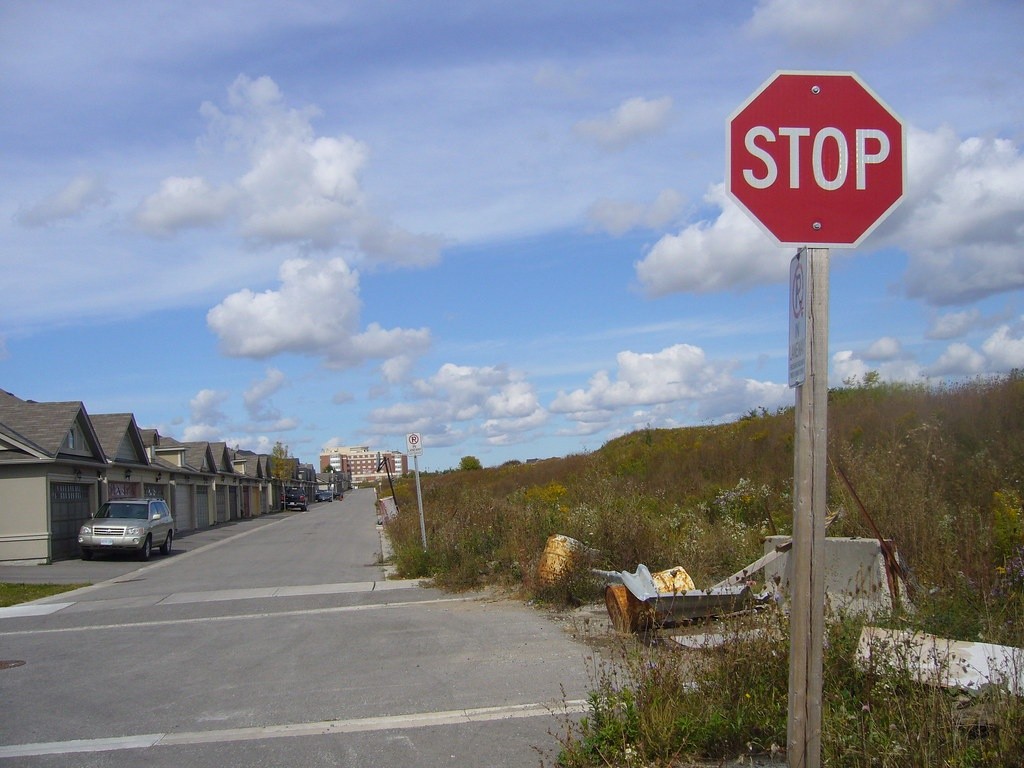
[280,488,309,512]
[77,497,174,561]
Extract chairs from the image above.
[143,506,155,516]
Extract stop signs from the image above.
[726,68,905,249]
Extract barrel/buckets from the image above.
[532,534,584,590]
[605,565,696,634]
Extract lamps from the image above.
[156,472,162,482]
[125,469,132,478]
[74,469,82,479]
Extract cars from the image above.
[316,490,344,502]
